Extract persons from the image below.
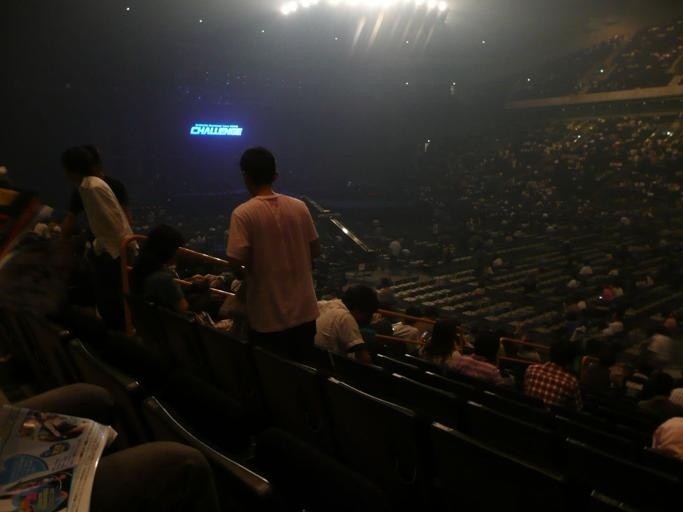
[1,2,682,510]
